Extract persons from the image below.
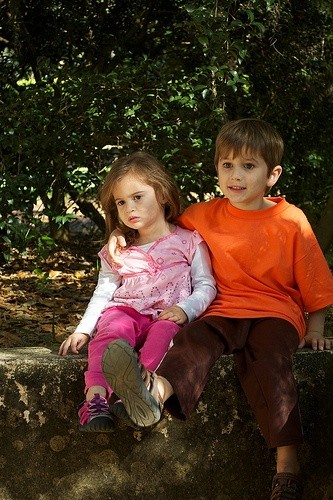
[107,118,333,500]
[58,152,217,433]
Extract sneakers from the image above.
[111,395,142,430]
[100,340,165,427]
[78,394,115,433]
[270,470,304,500]
